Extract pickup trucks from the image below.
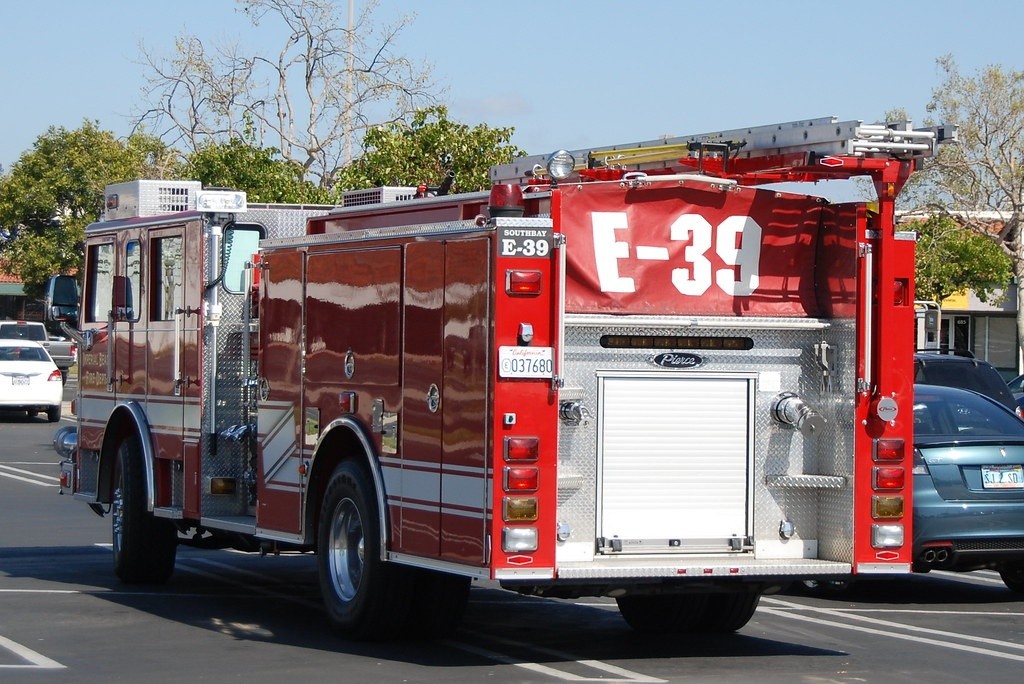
[0,320,77,386]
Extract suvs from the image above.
[915,345,1024,417]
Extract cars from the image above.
[913,383,1024,599]
[0,338,64,422]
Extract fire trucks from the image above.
[46,115,961,652]
